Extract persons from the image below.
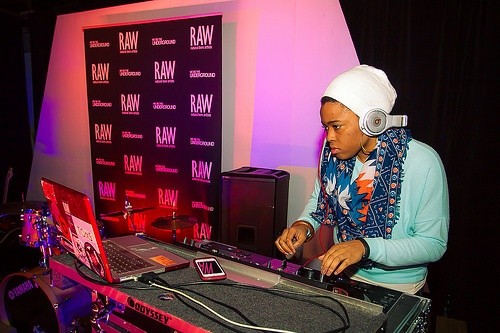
[274,65,451,296]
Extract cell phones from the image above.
[194,257,227,281]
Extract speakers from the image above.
[219,167,290,261]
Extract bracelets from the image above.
[291,220,316,243]
[354,237,370,261]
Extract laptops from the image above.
[40,176,190,284]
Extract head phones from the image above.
[359,107,407,137]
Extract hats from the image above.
[322,62,397,119]
